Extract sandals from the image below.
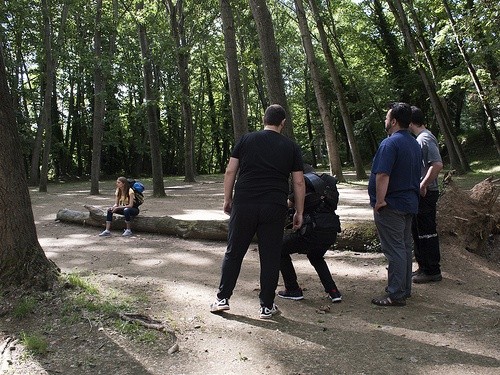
[371,294,406,306]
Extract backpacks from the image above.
[128,187,144,207]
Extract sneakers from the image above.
[209,297,230,312]
[278,287,304,300]
[257,303,280,319]
[327,290,343,302]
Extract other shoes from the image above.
[122,229,132,236]
[99,230,110,236]
[413,272,442,284]
[411,268,420,277]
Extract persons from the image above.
[275,172,343,302]
[368,102,422,307]
[406,106,443,284]
[99,176,140,236]
[209,104,306,318]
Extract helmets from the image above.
[133,182,145,193]
